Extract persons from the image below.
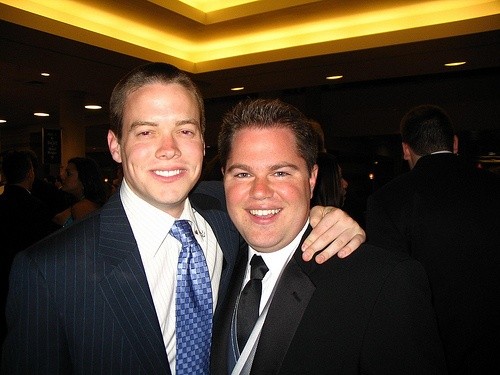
[0,62,366,375]
[0,99,500,375]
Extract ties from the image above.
[168,220,210,375]
[236,254,270,352]
